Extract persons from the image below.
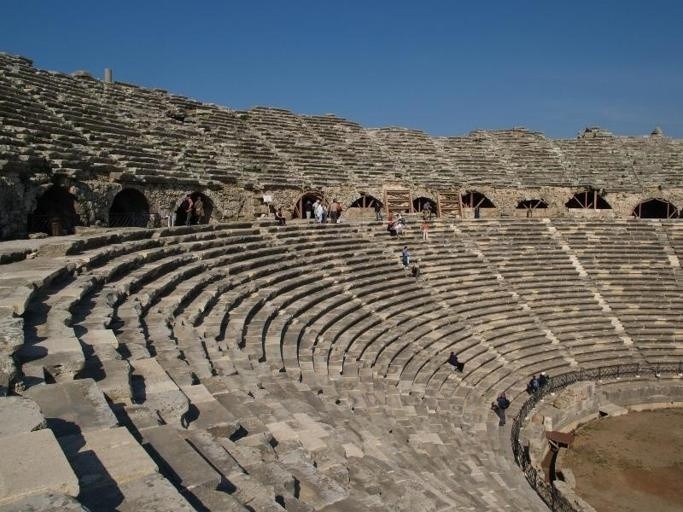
[528,374,539,392]
[401,246,407,266]
[386,201,432,241]
[495,390,510,428]
[539,370,548,385]
[273,207,286,226]
[193,196,206,225]
[182,194,194,227]
[410,263,417,277]
[302,197,343,224]
[375,199,383,221]
[448,350,465,373]
